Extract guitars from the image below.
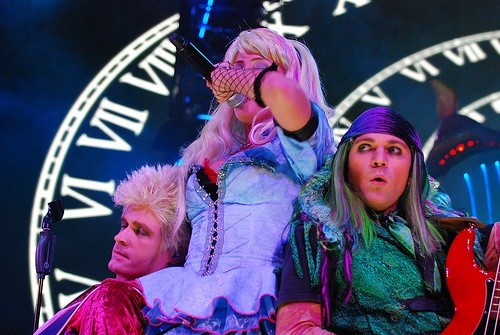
[442,227,500,335]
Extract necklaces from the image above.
[240,143,254,149]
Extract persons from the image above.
[136,27,336,335]
[275,106,500,335]
[33,164,191,335]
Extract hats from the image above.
[339,107,422,151]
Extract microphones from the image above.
[167,32,246,109]
[41,197,66,231]
